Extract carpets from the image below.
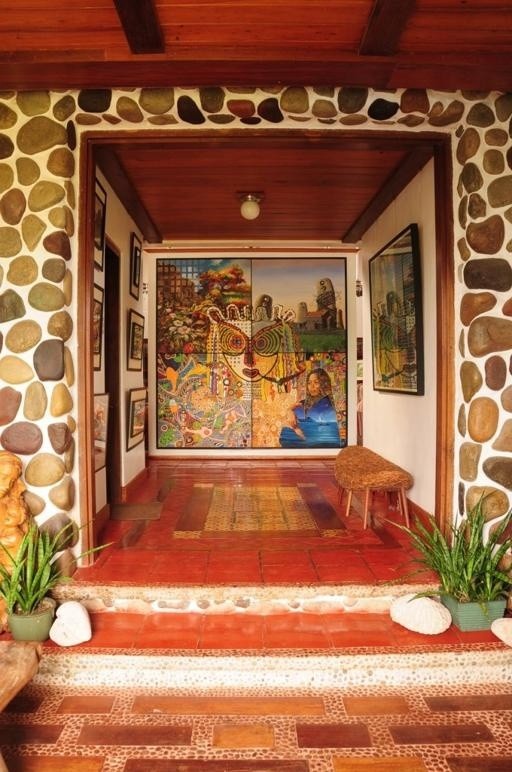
[108,503,164,520]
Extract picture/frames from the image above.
[156,257,348,449]
[94,177,108,272]
[127,308,144,374]
[128,231,143,301]
[128,386,148,452]
[93,283,106,373]
[92,394,111,472]
[368,222,423,396]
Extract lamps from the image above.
[240,191,263,221]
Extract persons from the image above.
[278,368,341,448]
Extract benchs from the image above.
[333,446,413,530]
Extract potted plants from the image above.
[379,487,512,632]
[0,519,117,643]
